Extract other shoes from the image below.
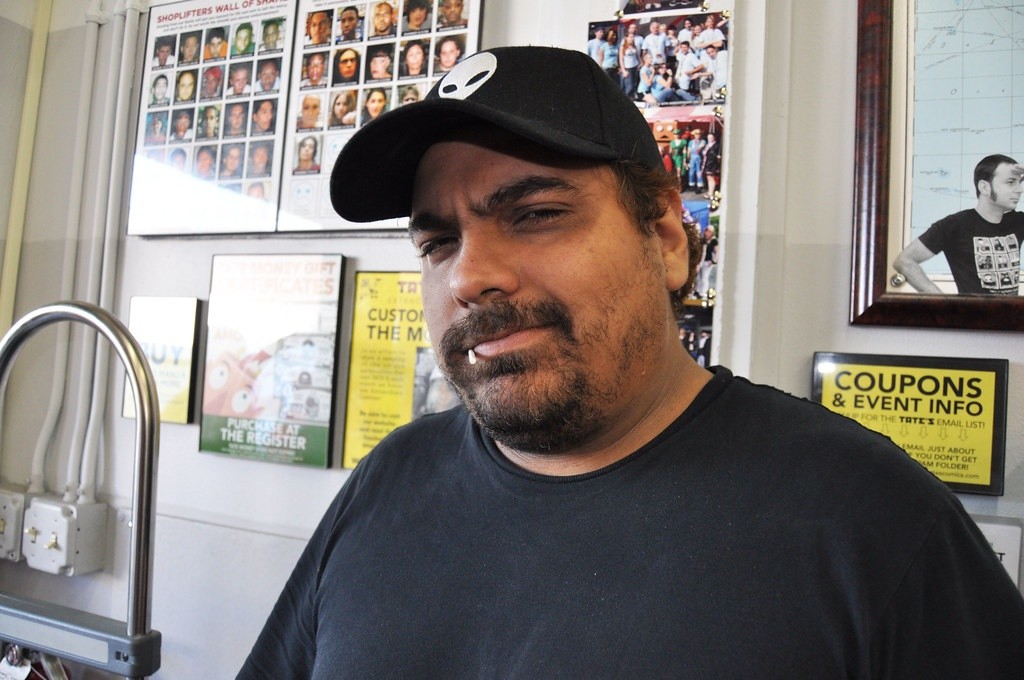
[695,187,702,193]
[704,193,715,200]
[688,186,696,191]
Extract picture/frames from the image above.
[848,0,1024,335]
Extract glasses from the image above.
[339,58,357,65]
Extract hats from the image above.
[204,67,221,81]
[690,129,705,135]
[672,129,681,134]
[706,225,715,231]
[328,47,666,222]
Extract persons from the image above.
[144,0,467,200]
[691,225,719,297]
[670,127,720,198]
[893,155,1024,295]
[587,15,727,101]
[235,46,1023,680]
[678,327,710,366]
